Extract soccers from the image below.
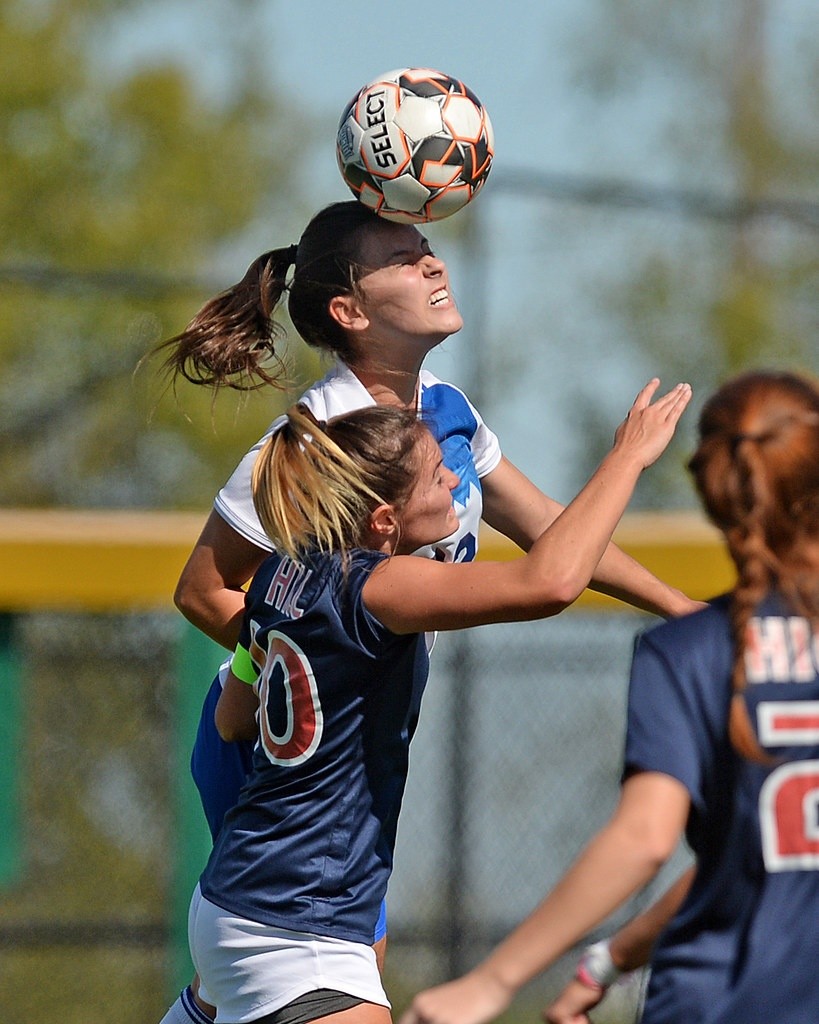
[332,65,496,231]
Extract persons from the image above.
[397,364,818,1024]
[132,206,704,1024]
[184,375,704,1024]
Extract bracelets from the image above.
[577,935,616,994]
